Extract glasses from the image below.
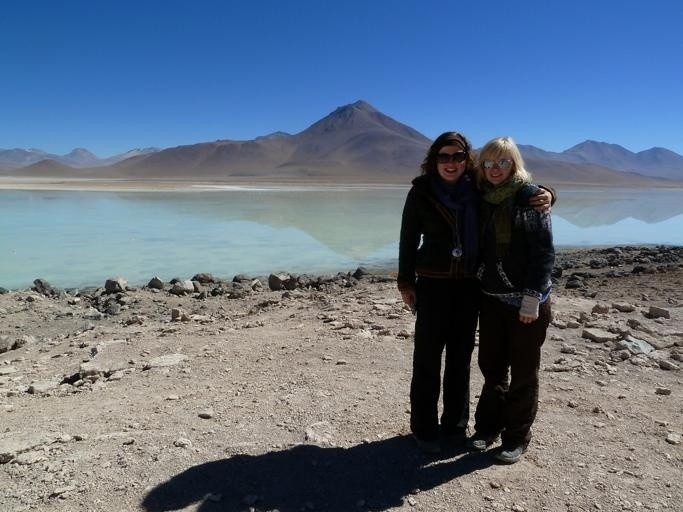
[483,158,514,170]
[436,151,468,165]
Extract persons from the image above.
[472,134,555,464]
[395,131,558,456]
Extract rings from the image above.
[539,199,544,204]
[545,199,548,204]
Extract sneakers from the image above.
[493,438,530,462]
[443,424,499,452]
[410,429,442,453]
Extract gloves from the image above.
[518,295,540,321]
